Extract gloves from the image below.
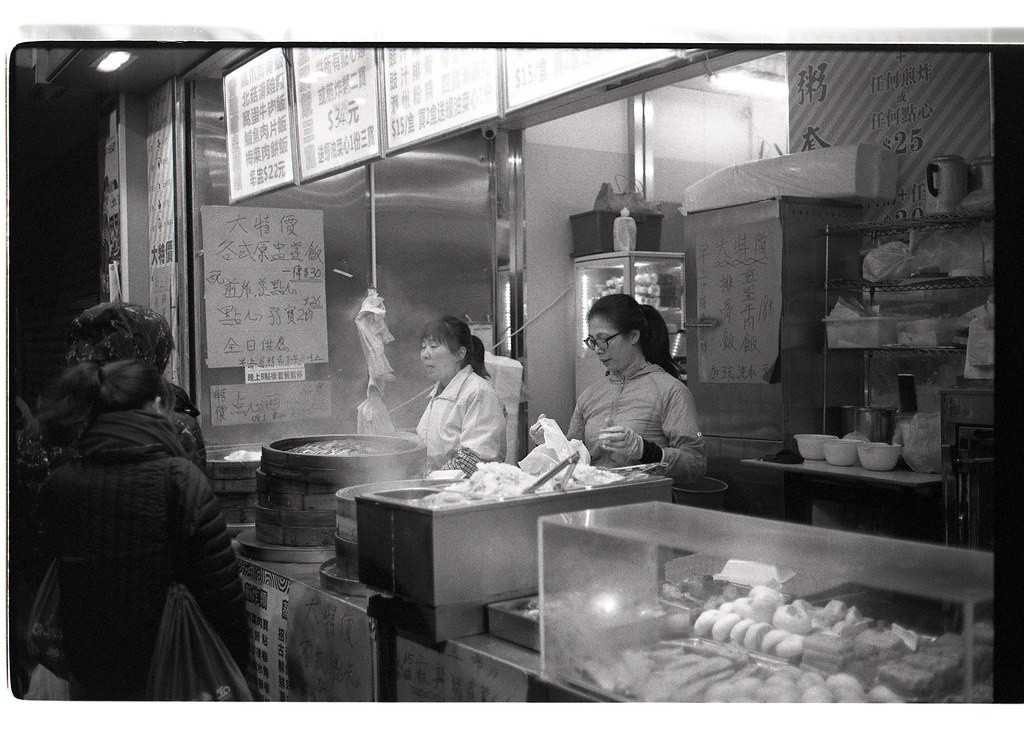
[529,414,545,446]
[598,426,643,460]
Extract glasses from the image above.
[583,331,623,351]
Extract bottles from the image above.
[612,207,636,251]
[600,272,679,306]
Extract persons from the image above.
[530,294,708,488]
[415,315,509,478]
[10,302,251,702]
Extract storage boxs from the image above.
[569,210,666,257]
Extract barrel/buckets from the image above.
[838,404,900,446]
[671,476,727,510]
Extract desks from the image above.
[739,458,945,534]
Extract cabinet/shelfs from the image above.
[822,212,996,444]
[540,501,995,704]
[938,385,996,548]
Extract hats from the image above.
[167,383,201,417]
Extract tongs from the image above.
[523,451,580,493]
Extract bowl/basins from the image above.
[794,434,838,461]
[856,442,901,472]
[820,439,861,466]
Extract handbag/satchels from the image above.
[144,584,253,702]
[26,556,70,682]
